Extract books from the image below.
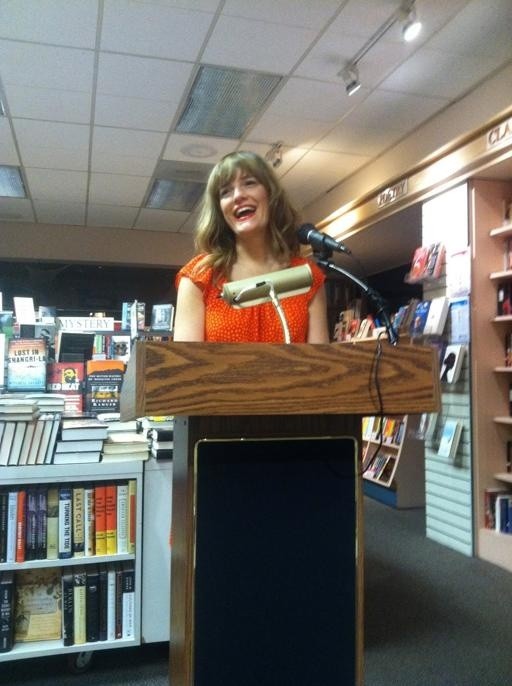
[484,486,510,533]
[336,298,423,484]
[2,561,135,653]
[0,291,172,465]
[1,478,136,563]
[495,283,511,368]
[406,244,464,461]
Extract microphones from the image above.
[296,223,352,255]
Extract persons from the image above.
[168,148,332,349]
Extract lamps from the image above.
[265,141,285,168]
[220,264,316,344]
[335,3,424,98]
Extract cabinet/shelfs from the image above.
[469,178,512,574]
[328,332,427,510]
